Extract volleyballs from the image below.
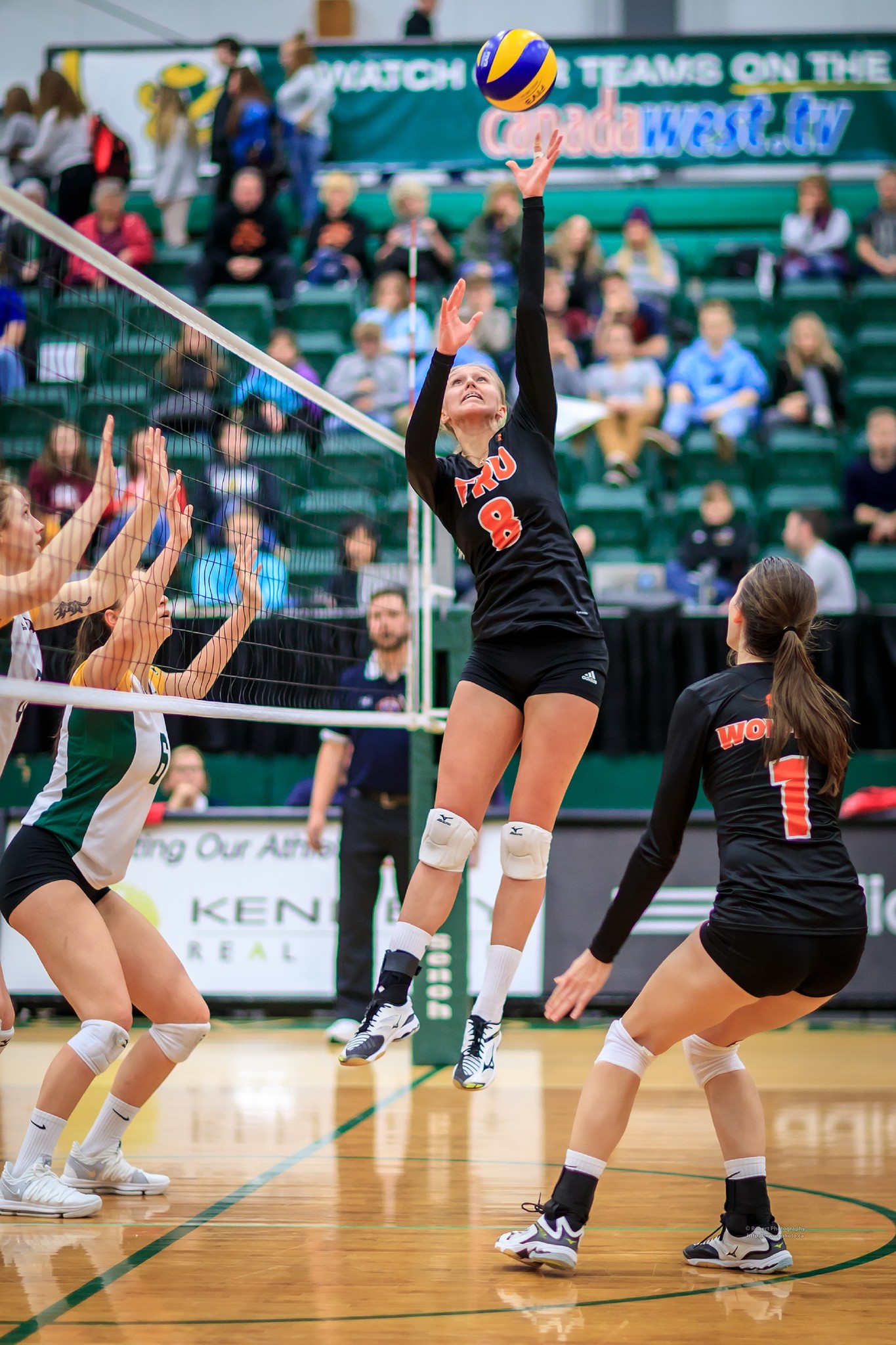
[476,28,558,112]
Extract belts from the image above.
[350,786,408,809]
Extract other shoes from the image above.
[326,1017,361,1044]
[605,451,644,488]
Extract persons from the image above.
[491,556,866,1274]
[0,2,896,1207]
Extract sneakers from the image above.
[452,1015,502,1091]
[63,1195,170,1246]
[681,1214,793,1274]
[494,1192,587,1272]
[338,995,420,1066]
[59,1141,170,1195]
[1,1153,102,1219]
[681,1262,793,1321]
[495,1276,585,1333]
[0,1213,101,1267]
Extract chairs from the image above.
[0,229,896,611]
